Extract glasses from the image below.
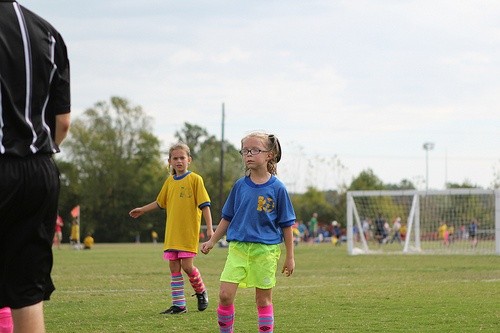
[240,147,270,156]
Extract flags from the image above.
[71,205,79,217]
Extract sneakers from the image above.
[159,306,187,314]
[196,291,208,311]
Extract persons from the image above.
[0,0,71,333]
[201,132,296,333]
[52,213,64,250]
[129,144,215,315]
[289,213,406,247]
[436,212,479,250]
[151,231,158,243]
[71,220,80,249]
[83,232,94,249]
